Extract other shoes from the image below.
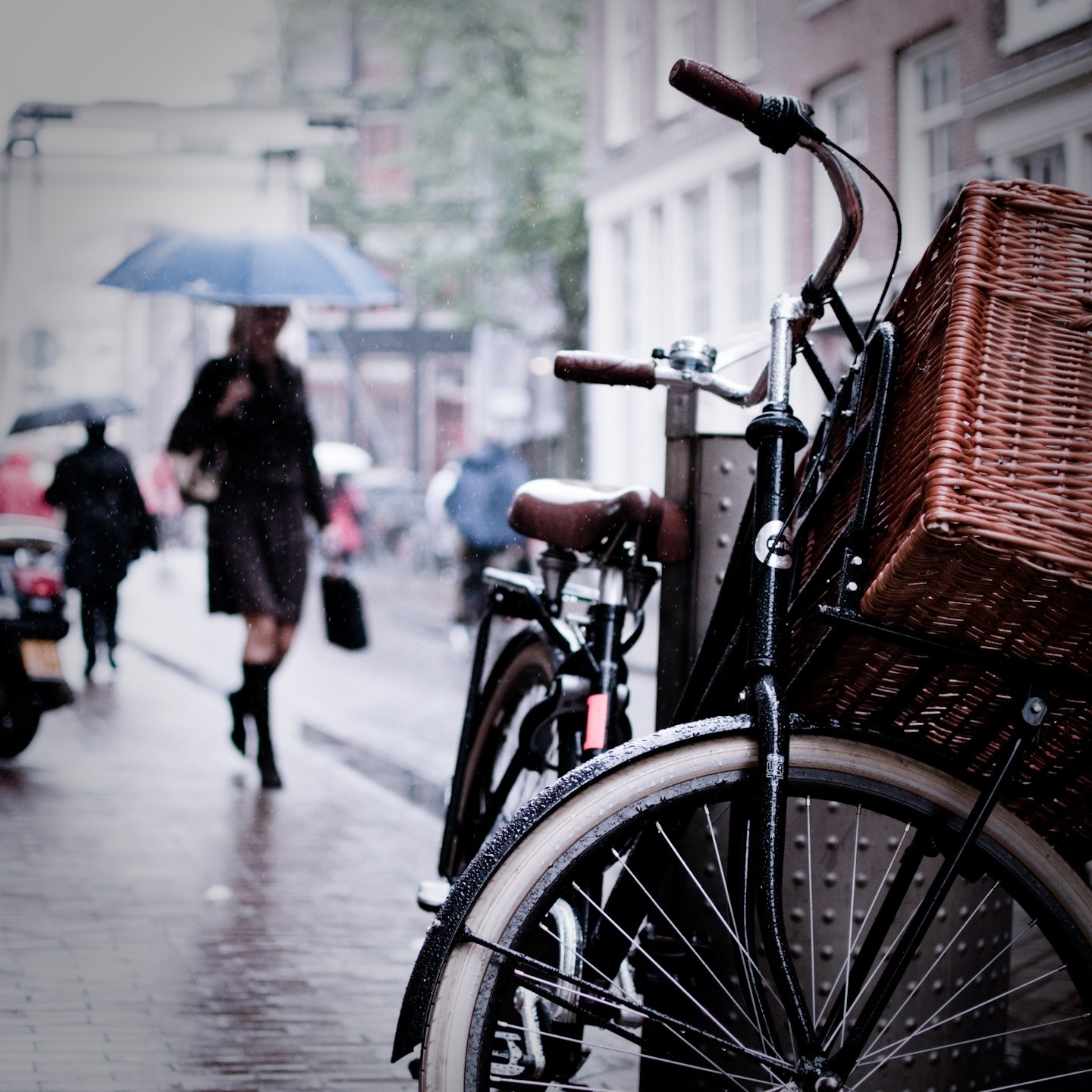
[257,755,281,788]
[230,691,251,753]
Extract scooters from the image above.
[1,510,78,766]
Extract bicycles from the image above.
[390,56,1091,1092]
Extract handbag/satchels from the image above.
[321,554,366,651]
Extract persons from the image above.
[166,304,369,790]
[42,417,162,677]
[316,474,367,565]
[418,436,534,633]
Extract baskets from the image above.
[779,176,1092,861]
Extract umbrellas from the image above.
[313,437,366,487]
[6,395,150,436]
[95,228,404,313]
